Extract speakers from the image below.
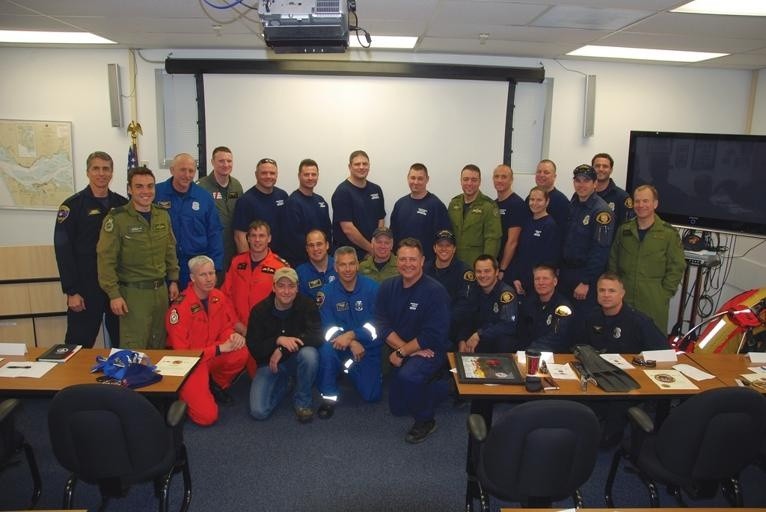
[584,74,597,137]
[107,62,124,128]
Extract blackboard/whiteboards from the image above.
[153,69,554,174]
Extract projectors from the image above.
[258,1,351,53]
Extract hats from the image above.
[372,226,393,238]
[573,164,597,181]
[273,267,298,285]
[434,230,455,242]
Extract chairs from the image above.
[465,397,601,512]
[605,386,766,508]
[0,420,43,509]
[43,384,193,512]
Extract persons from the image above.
[98,165,182,351]
[376,236,456,448]
[51,149,132,351]
[156,146,685,426]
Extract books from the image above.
[36,341,83,365]
[754,377,766,391]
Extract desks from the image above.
[446,351,766,398]
[0,344,205,399]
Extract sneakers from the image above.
[294,406,313,422]
[405,419,436,444]
[318,404,333,418]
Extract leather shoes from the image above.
[211,386,233,405]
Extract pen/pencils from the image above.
[7,366,31,368]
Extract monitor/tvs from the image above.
[624,129,766,239]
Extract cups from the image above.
[525,349,542,377]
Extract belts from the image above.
[119,279,165,290]
